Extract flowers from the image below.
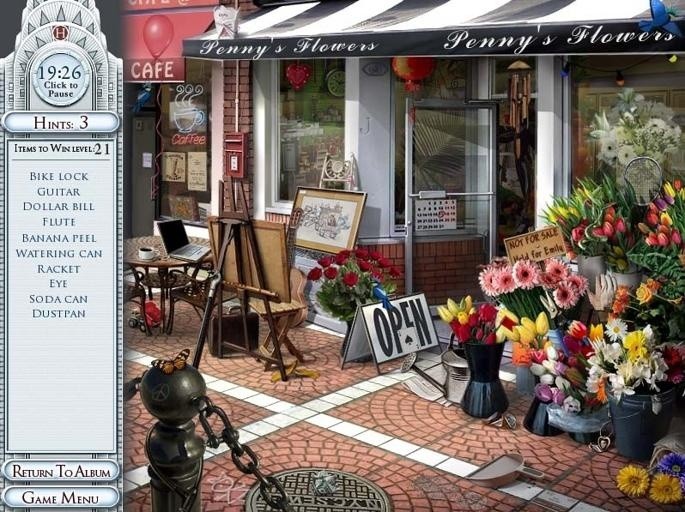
[589,88,682,205]
[436,172,684,416]
[616,449,685,505]
[306,246,403,322]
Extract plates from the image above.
[138,255,159,262]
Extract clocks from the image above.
[323,67,345,98]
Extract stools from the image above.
[248,299,307,372]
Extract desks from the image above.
[123,235,212,334]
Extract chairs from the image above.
[123,280,152,337]
[167,269,238,335]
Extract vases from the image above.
[523,377,567,437]
[568,405,607,445]
[340,317,373,363]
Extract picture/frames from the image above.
[287,185,367,256]
[208,216,291,303]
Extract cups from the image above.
[172,108,203,134]
[138,246,160,260]
